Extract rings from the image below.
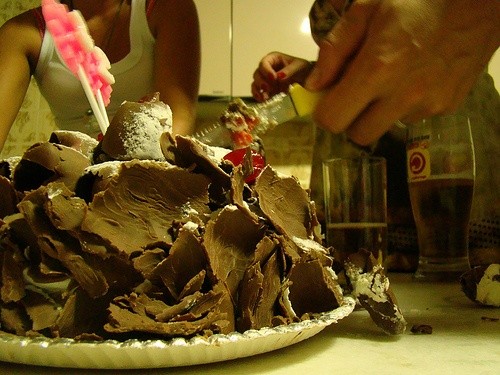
[395,120,407,129]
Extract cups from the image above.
[323,158,387,274]
[407,114,476,280]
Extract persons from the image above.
[0,0,201,163]
[252,0,500,272]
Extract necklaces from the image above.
[69,0,124,115]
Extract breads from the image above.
[459,264,500,307]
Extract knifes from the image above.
[192,83,321,147]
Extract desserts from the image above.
[0,93,406,340]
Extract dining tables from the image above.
[0,272,500,375]
